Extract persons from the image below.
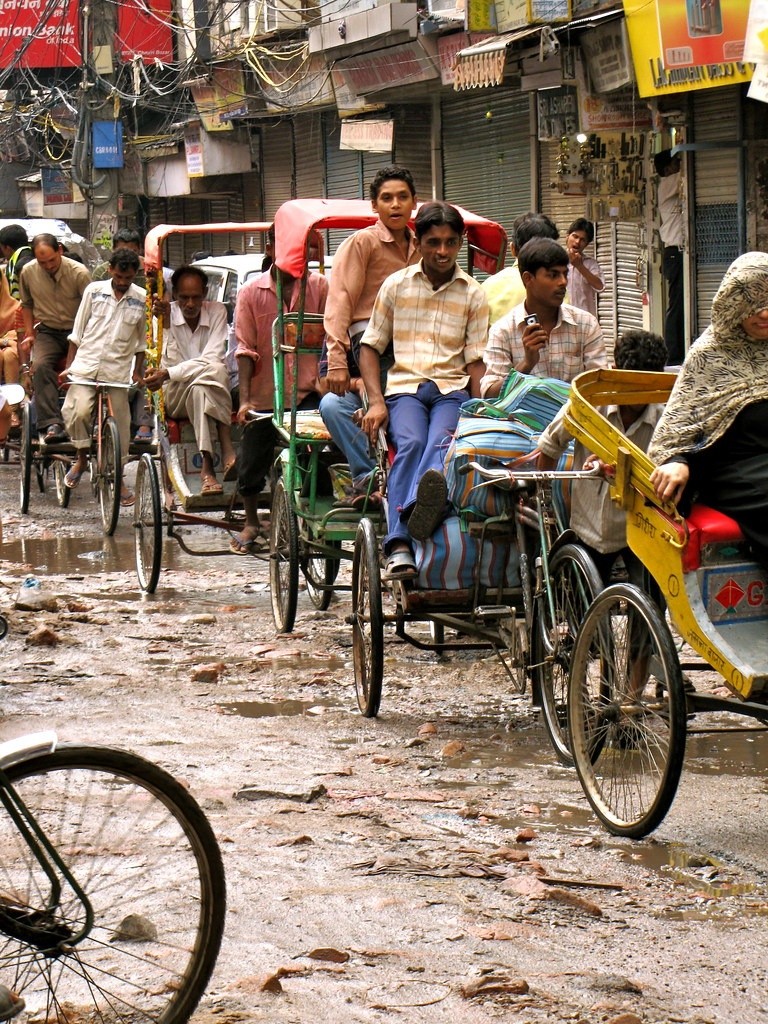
[645,251,768,583]
[360,201,490,580]
[143,265,241,497]
[323,166,423,401]
[1,152,686,746]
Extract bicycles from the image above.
[265,197,510,633]
[343,374,618,767]
[16,304,140,537]
[566,369,768,840]
[132,220,336,612]
[0,730,226,1024]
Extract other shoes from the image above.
[351,490,383,509]
[44,425,68,445]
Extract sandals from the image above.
[132,429,152,443]
[408,467,448,540]
[219,452,240,483]
[384,551,418,579]
[200,476,224,494]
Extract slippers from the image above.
[121,490,135,508]
[229,523,260,555]
[63,464,86,488]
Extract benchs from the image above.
[627,487,768,625]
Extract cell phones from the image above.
[524,313,540,333]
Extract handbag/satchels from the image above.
[413,368,629,588]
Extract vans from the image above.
[187,253,334,339]
